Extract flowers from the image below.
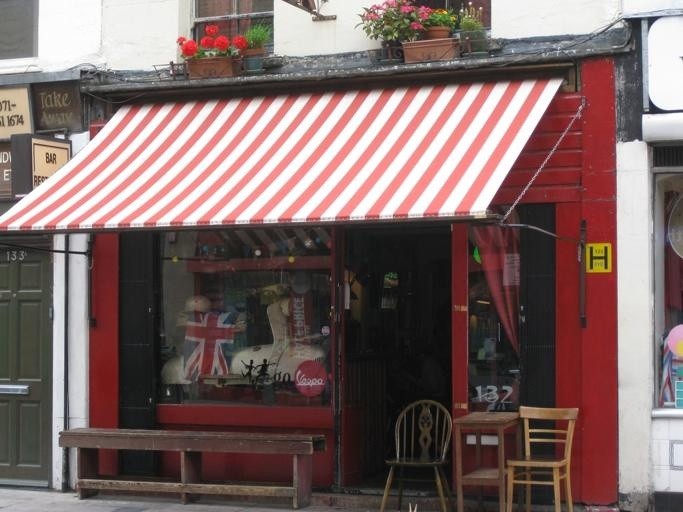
[355,0,484,39]
[175,23,249,59]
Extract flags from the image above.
[182,310,240,381]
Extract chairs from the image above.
[379,397,580,512]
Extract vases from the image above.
[239,19,271,58]
[187,57,244,78]
[399,37,456,64]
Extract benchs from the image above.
[57,427,329,508]
